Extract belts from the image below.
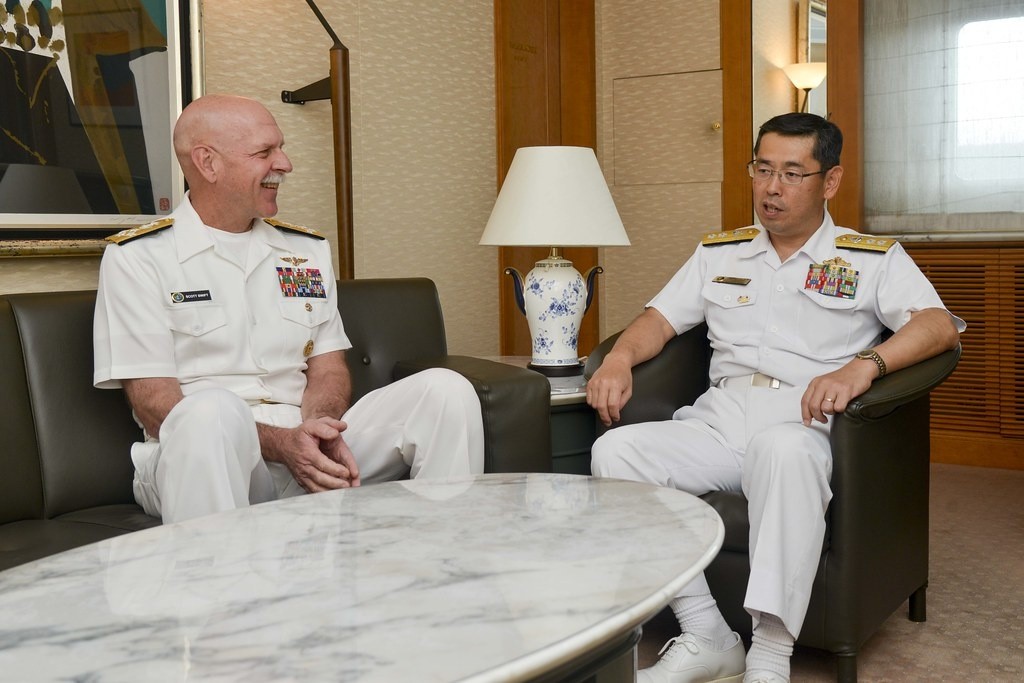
[724,373,794,389]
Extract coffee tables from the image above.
[0,472,725,683]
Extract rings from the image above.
[824,398,834,402]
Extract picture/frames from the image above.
[0,0,206,258]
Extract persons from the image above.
[585,112,967,683]
[92,96,484,523]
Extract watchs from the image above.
[856,350,886,378]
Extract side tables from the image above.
[479,356,619,477]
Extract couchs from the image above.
[584,318,963,683]
[0,278,552,574]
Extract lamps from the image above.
[784,62,827,113]
[478,145,630,379]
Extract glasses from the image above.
[747,160,826,185]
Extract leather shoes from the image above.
[635,632,746,682]
